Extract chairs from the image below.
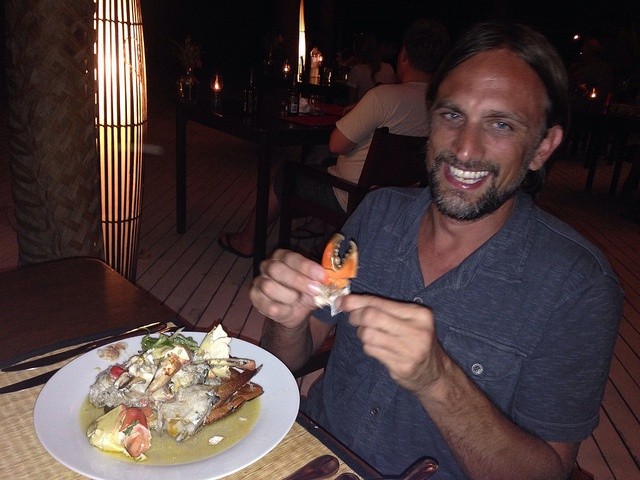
[295,127,596,480]
[278,127,429,249]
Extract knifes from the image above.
[0,326,176,394]
[1,321,167,371]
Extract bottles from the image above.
[242,69,258,119]
[287,72,299,115]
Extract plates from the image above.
[33,332,301,480]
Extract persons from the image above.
[251,18,624,480]
[345,37,397,102]
[217,19,452,259]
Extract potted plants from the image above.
[169,36,202,96]
